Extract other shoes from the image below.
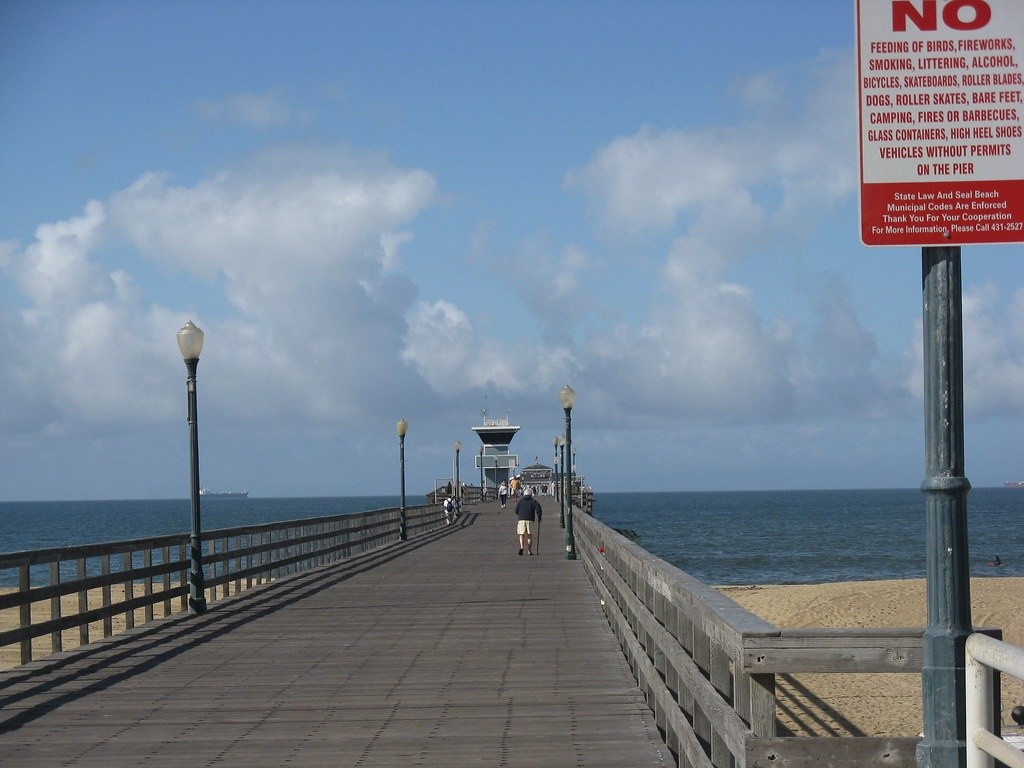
[501,504,506,508]
[528,553,533,555]
[449,523,451,526]
[519,548,524,555]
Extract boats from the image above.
[200,487,250,498]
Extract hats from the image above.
[524,489,533,495]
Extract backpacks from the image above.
[446,499,454,512]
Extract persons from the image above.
[498,482,508,508]
[511,484,548,502]
[443,495,456,525]
[515,488,542,556]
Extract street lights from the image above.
[396,418,408,541]
[559,433,565,529]
[478,445,484,502]
[553,435,559,502]
[176,321,208,616]
[454,440,462,516]
[572,448,576,476]
[494,454,498,499]
[559,385,577,561]
